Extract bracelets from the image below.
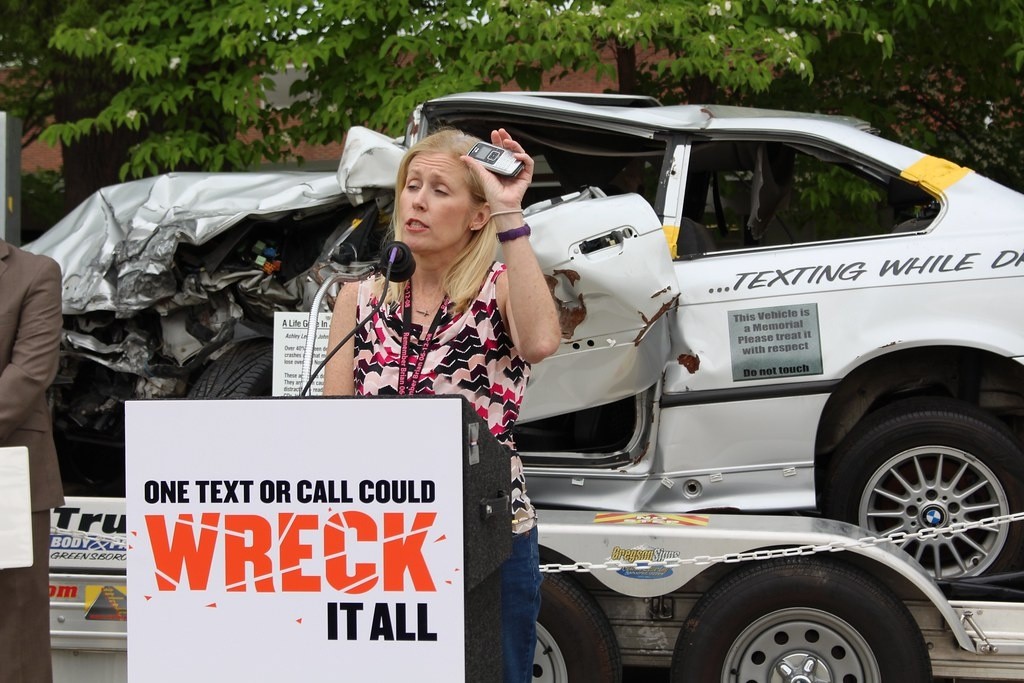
[490,209,523,217]
[495,221,531,243]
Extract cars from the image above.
[43,492,1024,683]
[16,89,1024,600]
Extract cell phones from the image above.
[467,142,525,178]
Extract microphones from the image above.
[380,241,416,283]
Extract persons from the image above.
[323,128,560,683]
[0,238,65,683]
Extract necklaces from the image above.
[411,282,443,316]
